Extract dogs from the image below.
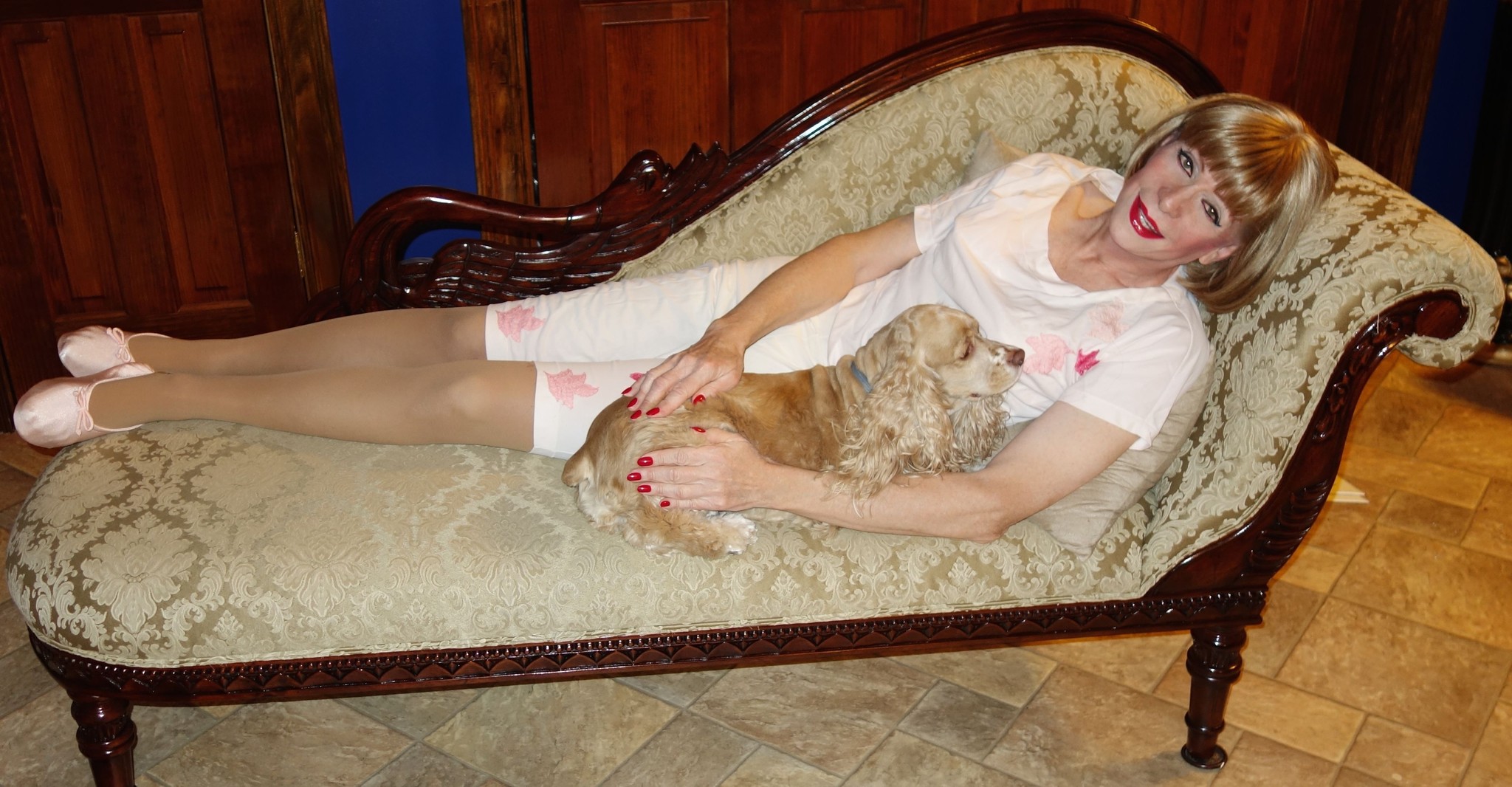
[562,304,1026,565]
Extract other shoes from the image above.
[57,326,172,380]
[13,362,155,448]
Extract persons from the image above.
[14,92,1345,545]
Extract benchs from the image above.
[4,11,1505,786]
